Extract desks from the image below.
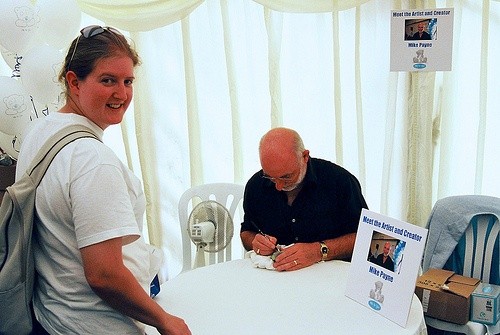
[142,260,427,335]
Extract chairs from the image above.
[417,194,500,335]
[178,183,245,273]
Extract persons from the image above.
[240,127,369,272]
[413,23,431,40]
[375,242,394,272]
[0,25,193,335]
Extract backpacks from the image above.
[0,124,104,335]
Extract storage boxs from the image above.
[470,283,500,325]
[414,269,480,325]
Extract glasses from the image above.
[68,25,122,72]
[263,173,293,181]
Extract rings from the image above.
[256,249,260,254]
[294,260,298,266]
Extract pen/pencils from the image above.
[252,221,278,251]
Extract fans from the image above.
[186,200,234,270]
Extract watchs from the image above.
[320,241,329,261]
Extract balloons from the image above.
[0,0,82,162]
